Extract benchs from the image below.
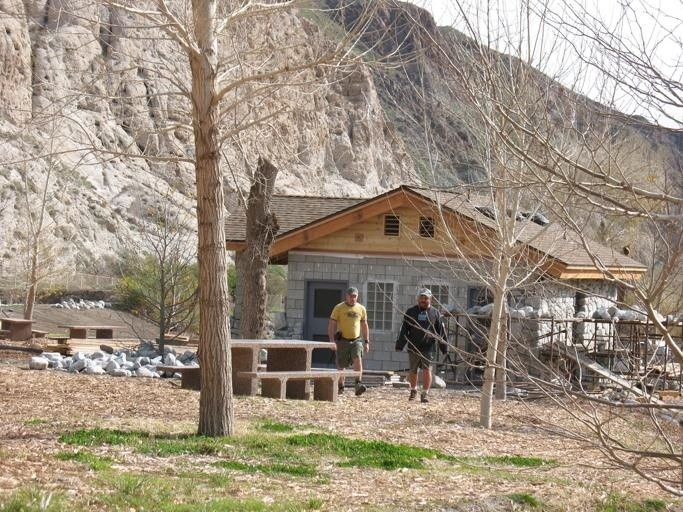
[156,364,363,401]
[0,330,48,339]
[61,325,127,338]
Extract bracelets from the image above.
[364,339,369,344]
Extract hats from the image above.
[346,287,359,296]
[416,288,432,297]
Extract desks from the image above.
[586,352,616,384]
[230,338,336,398]
[0,318,37,341]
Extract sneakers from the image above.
[409,390,417,400]
[421,393,428,402]
[338,384,345,394]
[355,383,366,395]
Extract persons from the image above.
[327,286,371,397]
[393,287,450,402]
